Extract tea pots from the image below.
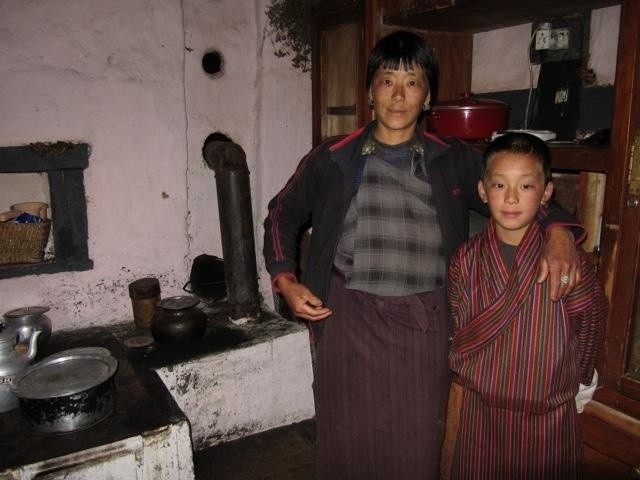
[0,323,43,413]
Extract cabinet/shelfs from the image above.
[458,139,608,354]
[455,383,639,479]
[315,5,370,134]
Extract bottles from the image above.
[129,278,161,330]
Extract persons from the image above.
[446,129,612,480]
[265,28,590,479]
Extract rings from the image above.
[561,275,570,284]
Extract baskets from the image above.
[0,219,51,263]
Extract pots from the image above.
[150,294,208,351]
[426,91,512,141]
[11,346,118,437]
[3,306,52,345]
